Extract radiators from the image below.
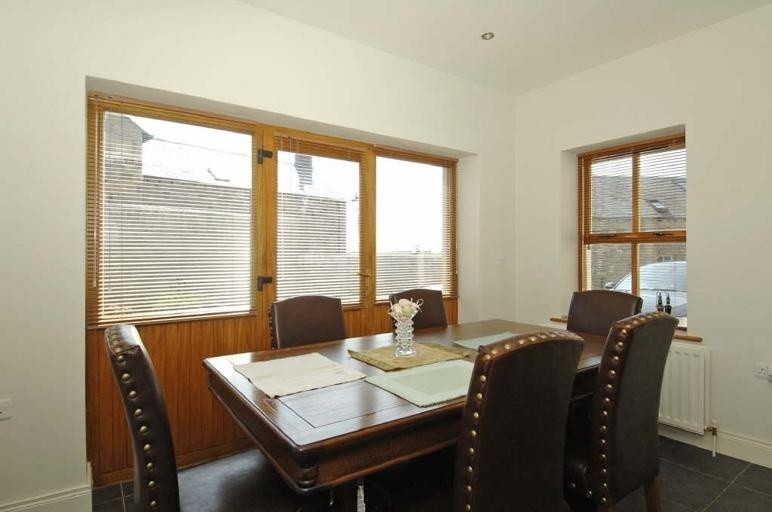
[656,341,712,436]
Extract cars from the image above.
[605,261,688,329]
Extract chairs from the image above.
[271,296,345,349]
[388,289,679,512]
[100,323,332,510]
